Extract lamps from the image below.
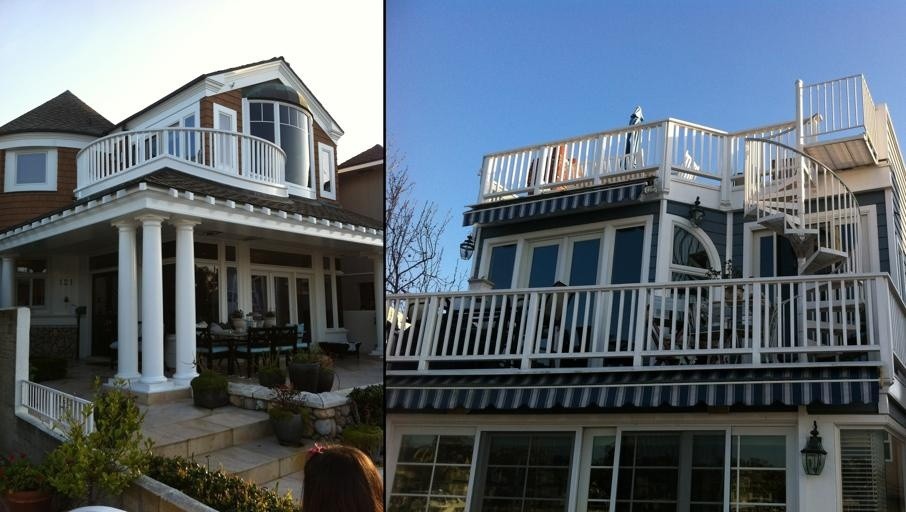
[460,233,475,260]
[800,420,827,475]
[691,196,706,227]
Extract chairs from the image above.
[409,281,574,367]
[195,321,312,379]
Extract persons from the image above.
[296,444,383,511]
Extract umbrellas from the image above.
[621,105,645,168]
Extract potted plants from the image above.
[267,384,309,447]
[191,368,229,408]
[347,384,385,465]
[254,345,287,388]
[285,338,340,393]
